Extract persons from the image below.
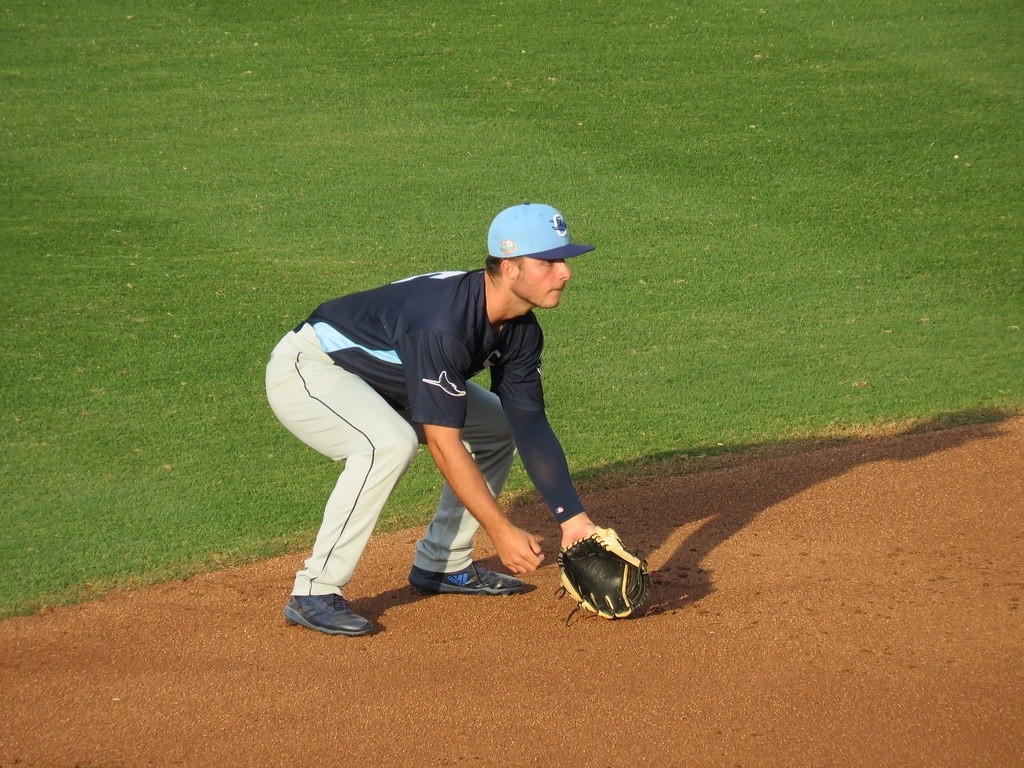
[264,202,646,633]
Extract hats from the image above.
[488,202,596,261]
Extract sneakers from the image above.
[283,593,373,636]
[409,563,525,597]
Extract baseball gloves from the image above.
[555,526,650,628]
